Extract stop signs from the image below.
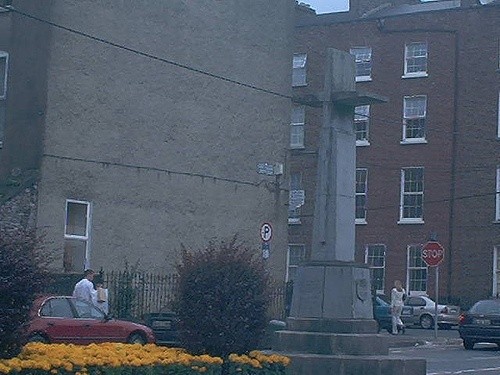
[419,241,446,267]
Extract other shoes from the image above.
[391,333,398,336]
[402,326,405,334]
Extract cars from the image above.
[371,293,402,335]
[15,293,157,350]
[394,294,459,328]
[457,296,500,350]
[147,295,183,347]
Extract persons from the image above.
[391,280,406,336]
[71,268,108,319]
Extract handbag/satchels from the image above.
[402,288,407,301]
